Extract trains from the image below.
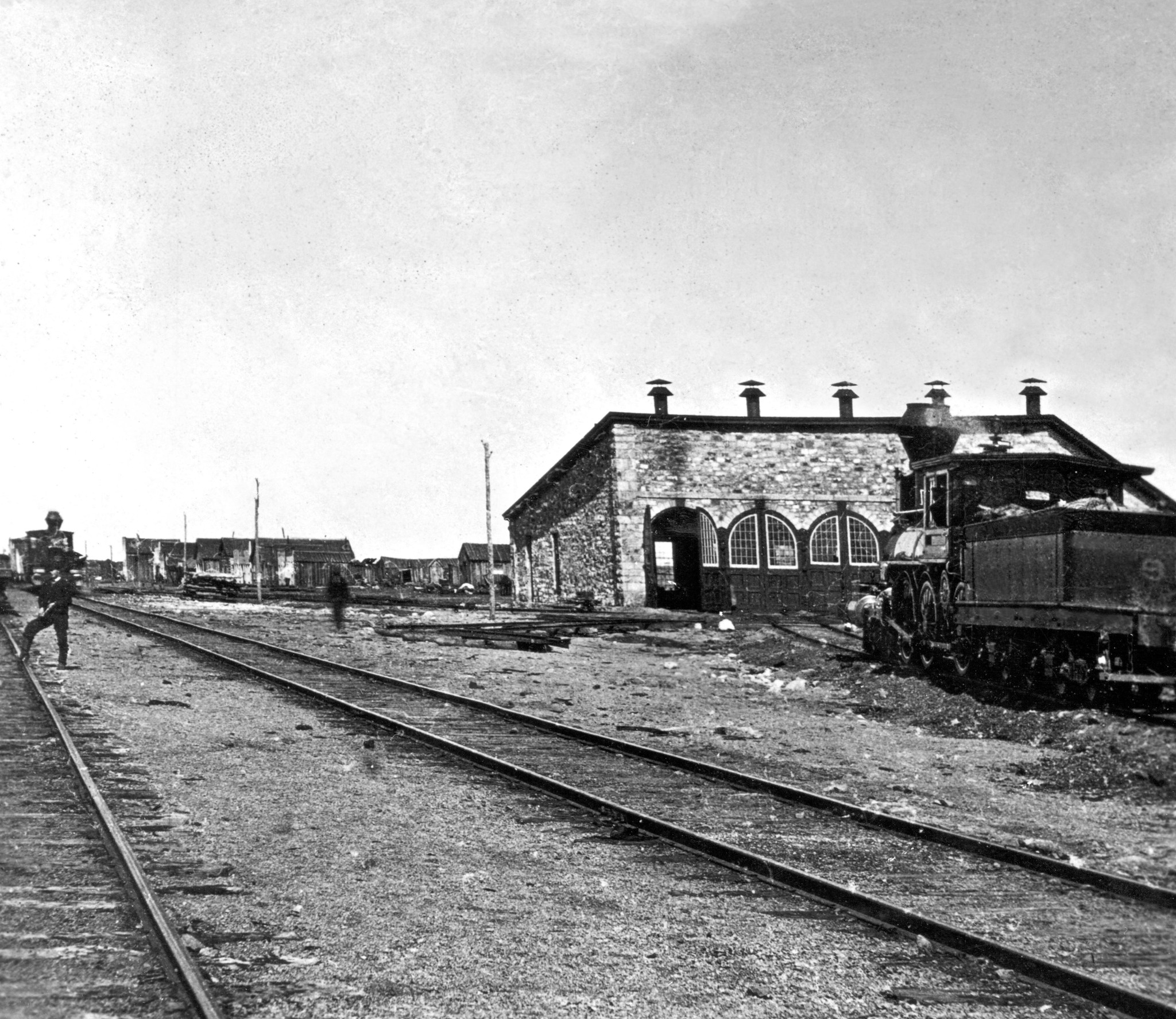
[856,420,1176,725]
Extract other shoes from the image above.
[12,650,30,664]
[57,662,66,670]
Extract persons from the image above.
[15,548,75,671]
[325,565,351,627]
